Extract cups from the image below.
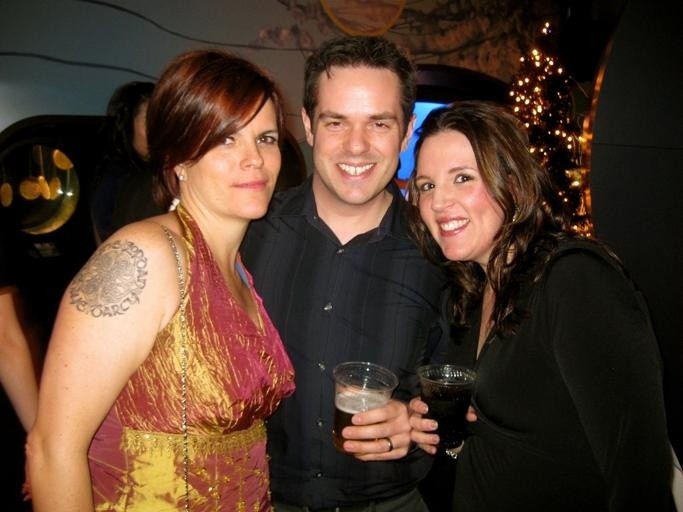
[412,365,475,449]
[327,362,397,454]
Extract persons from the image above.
[1,33,683,511]
